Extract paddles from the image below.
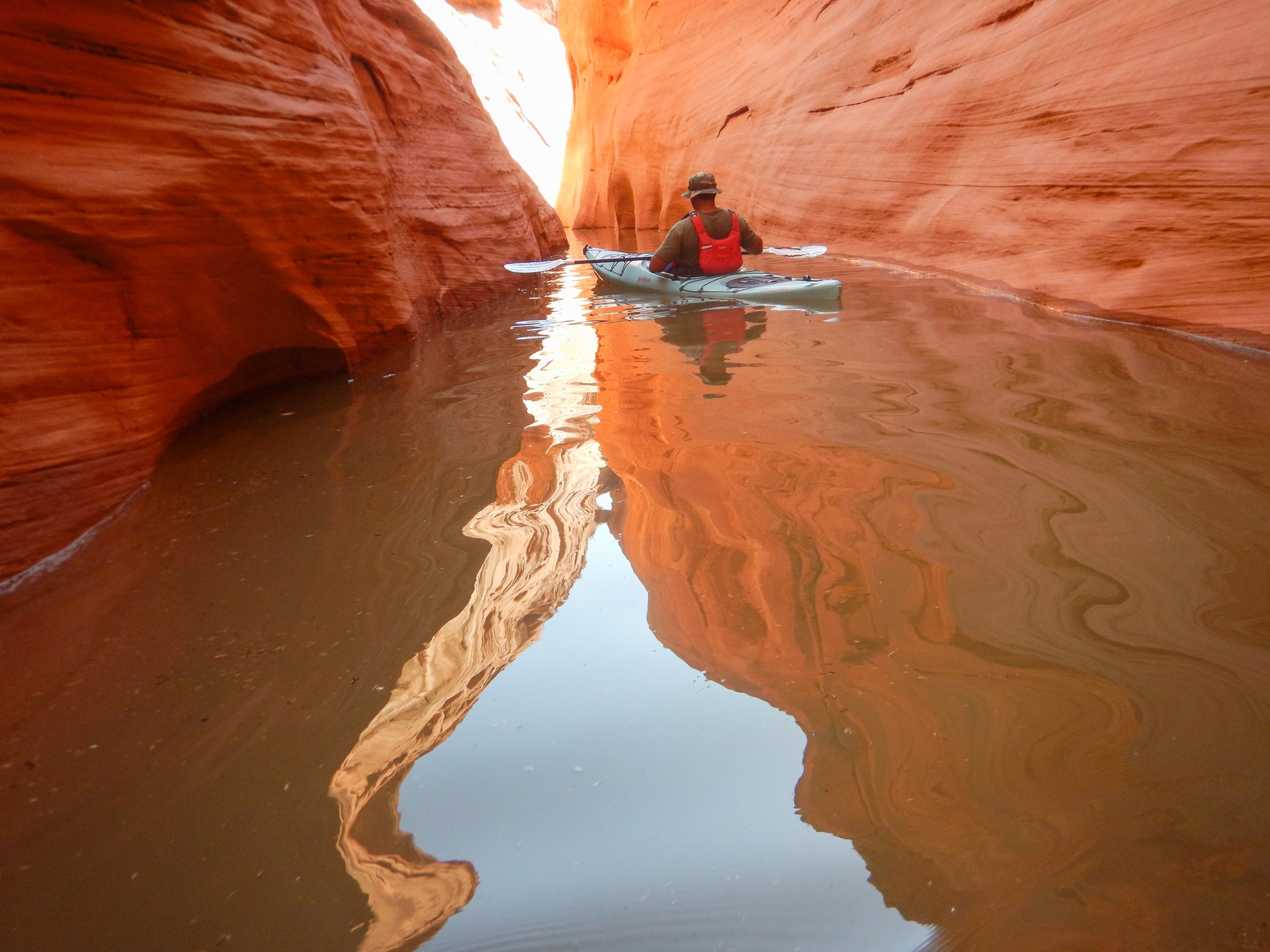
[503,245,827,274]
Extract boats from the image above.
[583,244,843,300]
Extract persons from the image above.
[649,172,763,277]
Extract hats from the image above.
[692,370,735,385]
[681,172,723,199]
